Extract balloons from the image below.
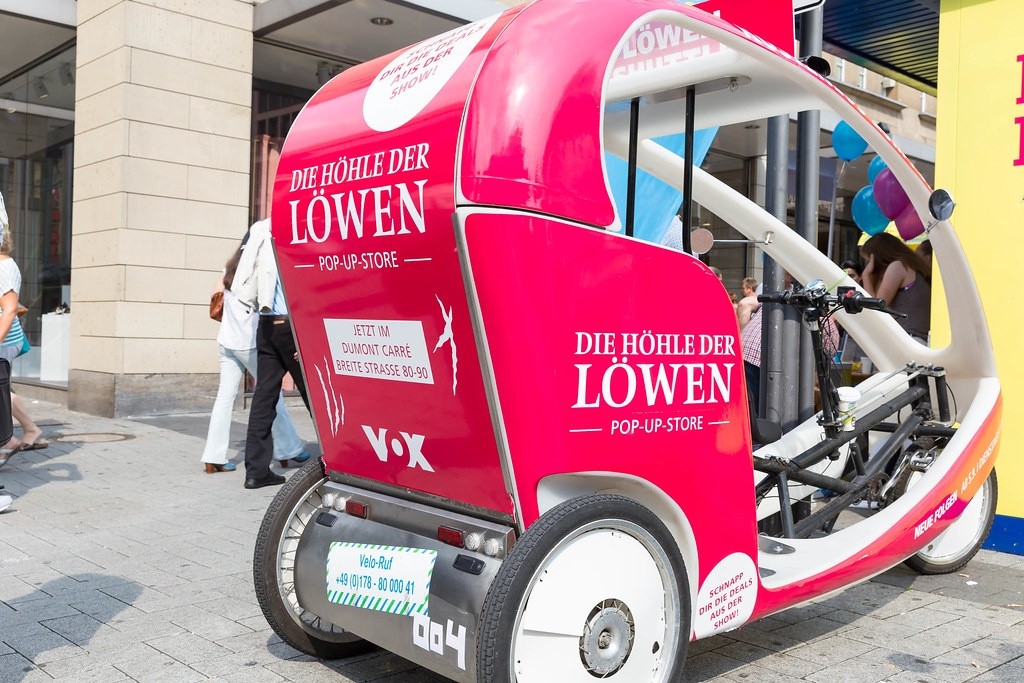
[831,120,868,163]
[851,154,924,241]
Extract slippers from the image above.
[19,442,49,451]
[0,440,23,468]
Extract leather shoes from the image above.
[244,470,286,489]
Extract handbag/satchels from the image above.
[210,292,223,323]
[16,335,31,357]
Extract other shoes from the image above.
[0,496,12,512]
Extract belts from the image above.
[260,315,289,320]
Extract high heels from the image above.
[206,462,237,473]
[280,452,311,467]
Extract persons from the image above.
[245,242,331,490]
[0,191,49,514]
[915,240,931,266]
[200,230,312,475]
[705,258,865,439]
[862,233,932,348]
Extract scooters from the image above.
[253,1,1010,683]
